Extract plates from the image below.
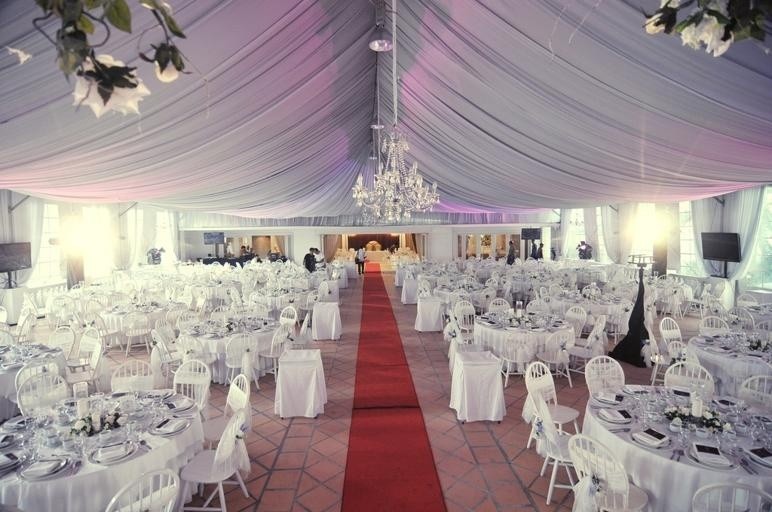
[186,315,275,338]
[0,390,198,480]
[594,387,771,472]
[693,331,771,363]
[479,309,567,331]
[0,341,60,369]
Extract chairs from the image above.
[396,256,772,512]
[0,259,348,512]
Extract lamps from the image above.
[368,150,378,160]
[368,0,393,52]
[352,1,439,224]
[368,98,385,129]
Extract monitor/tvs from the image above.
[700,233,741,262]
[521,229,542,241]
[204,232,224,245]
[0,244,31,273]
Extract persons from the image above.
[303,248,316,272]
[356,246,367,274]
[507,238,544,264]
[240,246,246,256]
[314,248,325,269]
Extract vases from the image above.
[1,0,193,117]
[643,0,771,58]
